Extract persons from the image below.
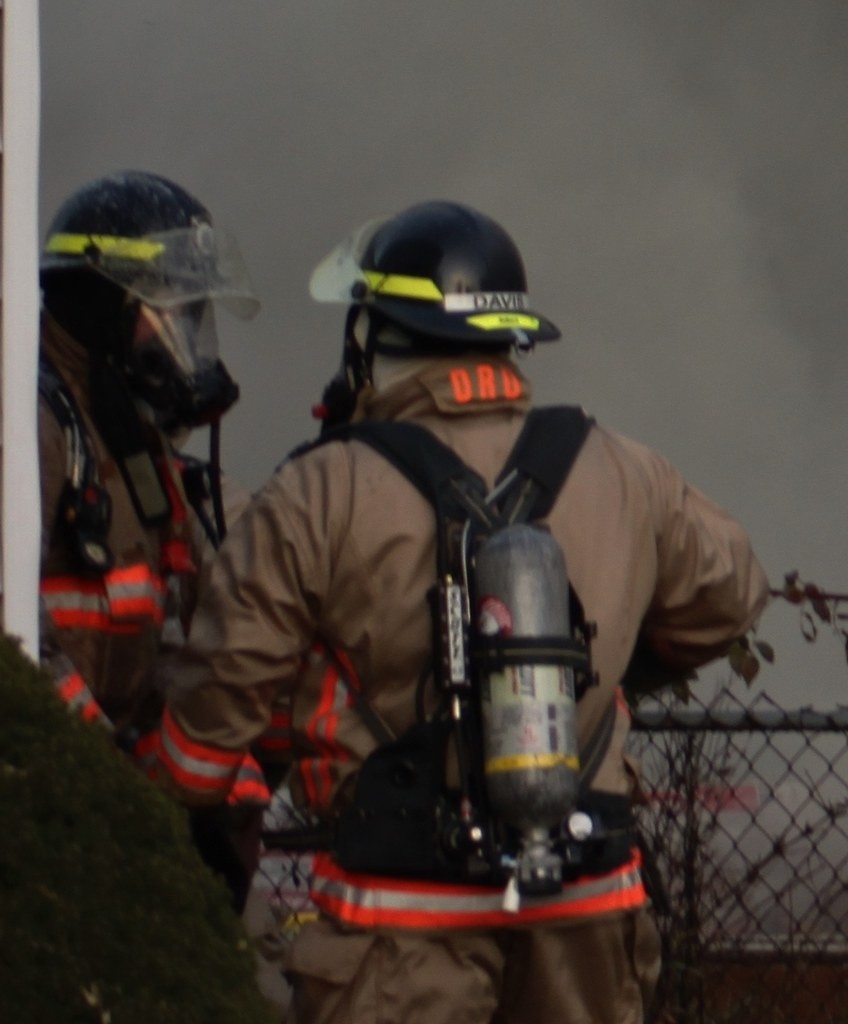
[39,173,292,923]
[152,201,771,1024]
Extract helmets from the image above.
[349,199,560,344]
[39,172,219,285]
